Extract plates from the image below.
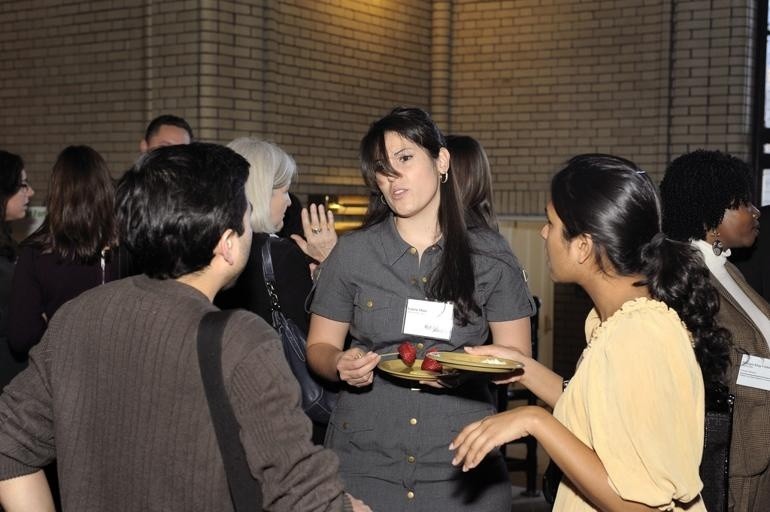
[424,351,526,374]
[375,358,454,383]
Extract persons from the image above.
[307,106,534,512]
[2,113,368,510]
[444,149,769,511]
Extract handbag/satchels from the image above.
[262,235,340,422]
[541,376,734,512]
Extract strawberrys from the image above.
[398,341,417,367]
[421,347,443,371]
